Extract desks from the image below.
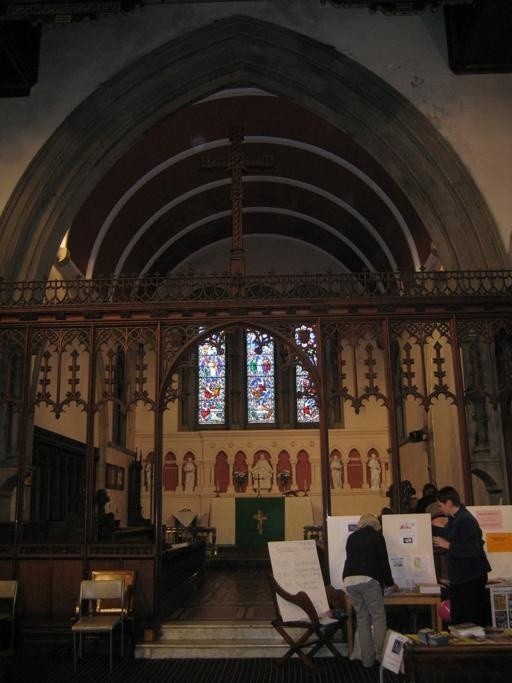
[381,629,511,682]
[163,540,205,611]
[333,585,442,661]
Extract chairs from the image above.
[89,572,136,619]
[0,580,19,661]
[264,539,347,672]
[70,580,125,674]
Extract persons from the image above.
[433,485,493,632]
[411,481,451,582]
[249,452,273,492]
[328,453,342,487]
[366,450,382,489]
[341,512,400,669]
[183,455,196,492]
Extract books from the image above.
[448,621,487,639]
